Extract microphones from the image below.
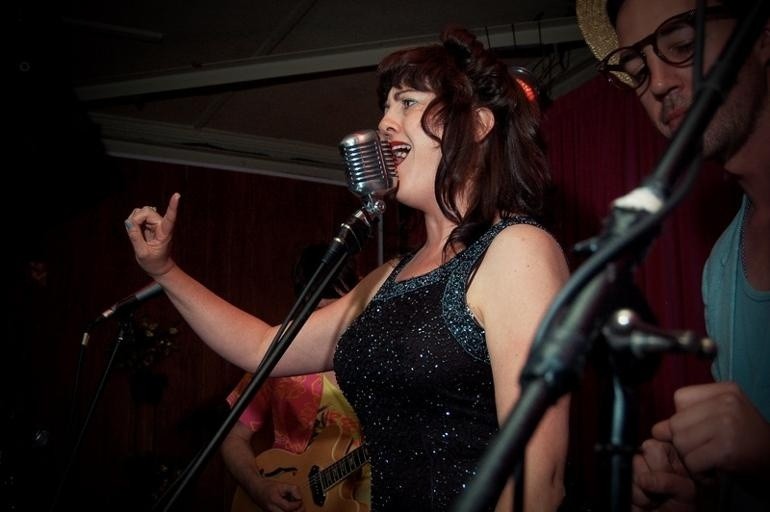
[338,129,399,214]
[95,280,162,321]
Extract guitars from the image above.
[232,424,370,512]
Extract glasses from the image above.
[593,5,733,90]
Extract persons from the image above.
[565,1,770,509]
[123,21,574,510]
[215,244,376,508]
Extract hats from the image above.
[573,0,638,89]
